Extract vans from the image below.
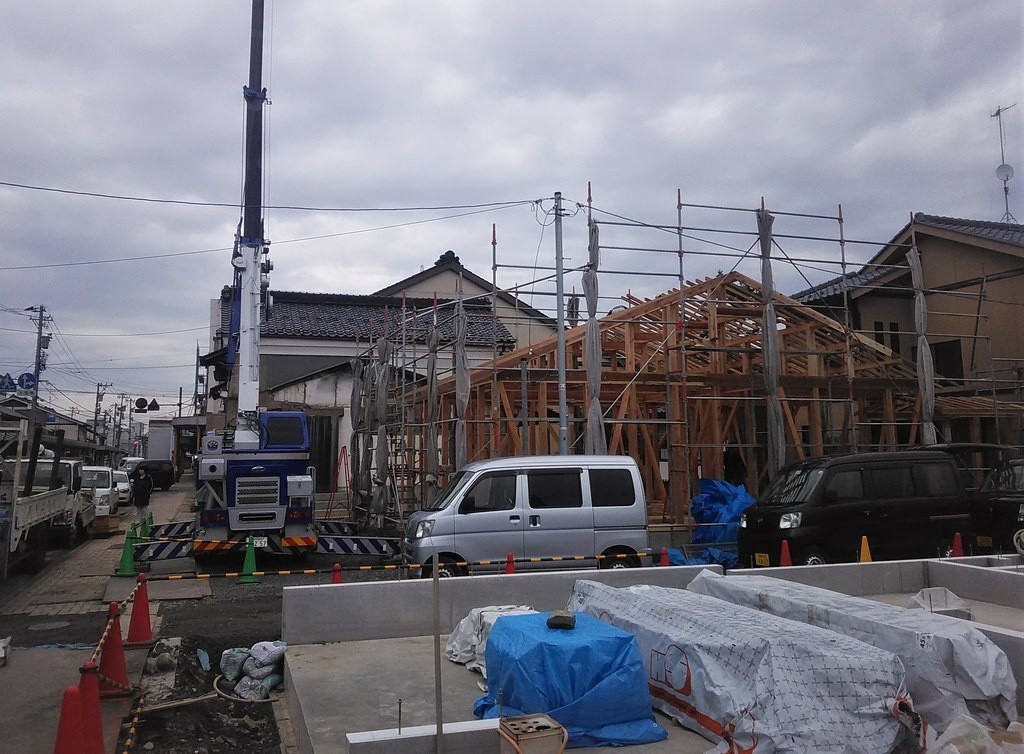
[962,453,1024,556]
[122,459,177,492]
[740,450,965,568]
[403,453,654,579]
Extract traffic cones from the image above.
[500,551,517,572]
[656,547,675,567]
[52,685,87,754]
[776,538,795,565]
[236,535,261,584]
[857,533,876,564]
[951,533,967,555]
[118,511,155,576]
[330,562,344,582]
[98,602,133,702]
[122,572,158,651]
[79,663,102,754]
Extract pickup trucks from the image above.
[80,466,121,516]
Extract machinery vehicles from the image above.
[192,0,317,572]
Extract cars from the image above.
[110,470,134,505]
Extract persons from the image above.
[130,464,153,527]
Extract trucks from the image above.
[4,459,97,545]
